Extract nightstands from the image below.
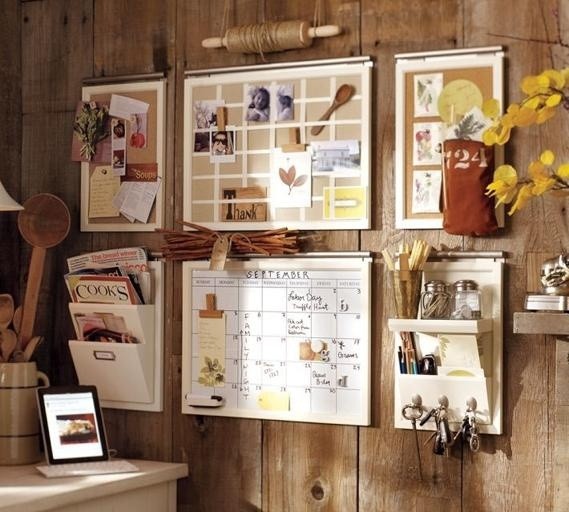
[0,458,192,512]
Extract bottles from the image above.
[451,279,482,320]
[420,280,453,320]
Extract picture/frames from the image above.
[180,54,378,232]
[390,52,504,229]
[79,75,175,233]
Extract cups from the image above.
[389,270,422,319]
[0,362,51,467]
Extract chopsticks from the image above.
[381,240,433,269]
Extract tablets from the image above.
[35,384,111,466]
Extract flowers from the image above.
[474,64,569,217]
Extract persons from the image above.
[213,131,232,154]
[246,85,272,122]
[277,88,293,119]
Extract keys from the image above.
[400,402,480,484]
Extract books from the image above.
[63,243,153,344]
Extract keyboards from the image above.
[36,460,139,479]
